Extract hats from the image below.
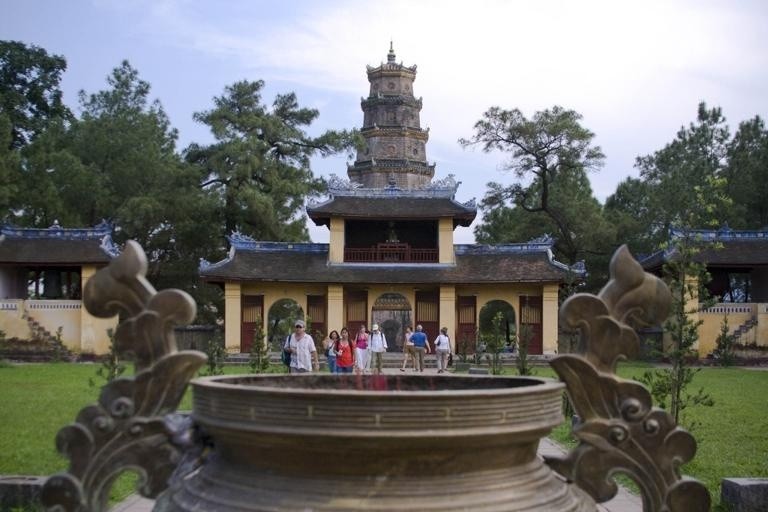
[294,319,305,330]
[442,326,447,333]
[372,324,378,331]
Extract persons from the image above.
[399,324,450,373]
[326,324,389,372]
[283,320,319,373]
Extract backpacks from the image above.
[281,347,290,366]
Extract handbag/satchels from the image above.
[448,353,452,365]
[325,348,329,356]
